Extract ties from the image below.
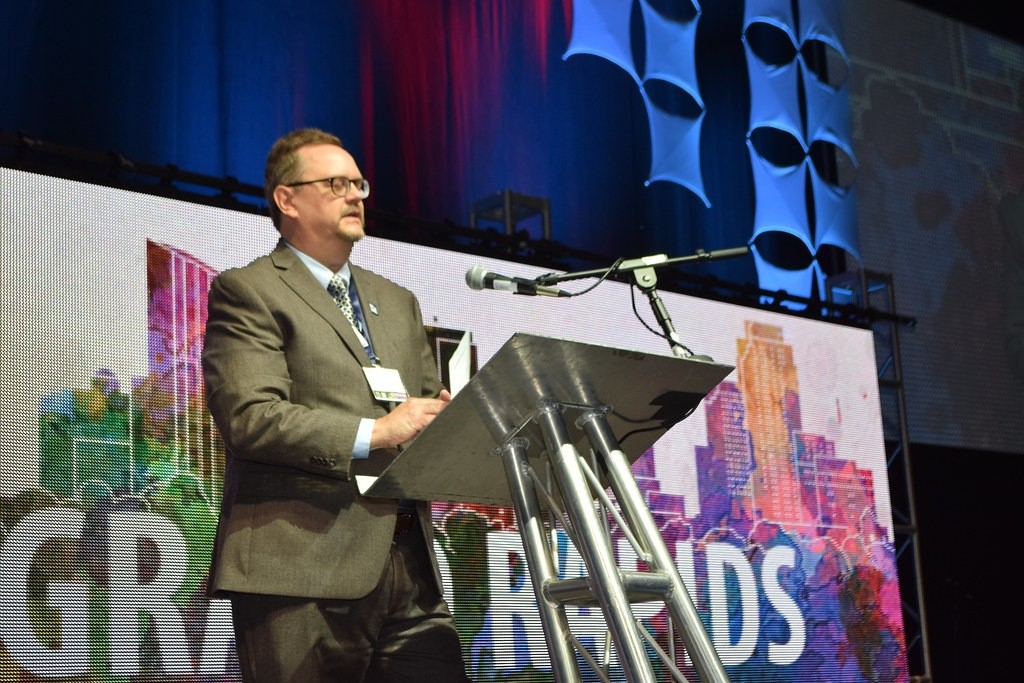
[328,275,357,333]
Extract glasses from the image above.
[285,176,370,200]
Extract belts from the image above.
[392,514,419,533]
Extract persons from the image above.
[202,127,471,683]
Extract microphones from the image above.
[465,267,571,298]
